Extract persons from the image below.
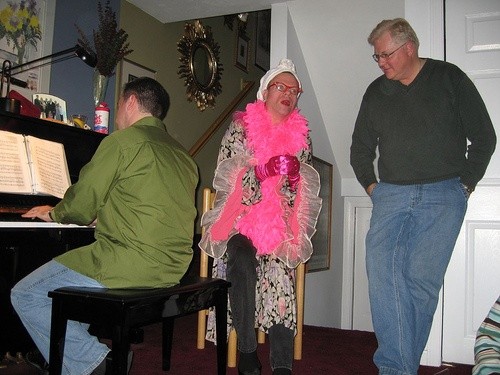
[197,59,324,372]
[348,17,500,375]
[34,93,64,121]
[10,77,199,375]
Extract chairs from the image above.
[197,188,304,368]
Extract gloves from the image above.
[287,153,301,192]
[254,154,296,182]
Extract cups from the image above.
[0,97,21,114]
[72,114,88,128]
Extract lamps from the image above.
[0,44,98,115]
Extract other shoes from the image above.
[26,349,51,375]
[273,367,291,375]
[238,350,261,375]
[103,350,134,375]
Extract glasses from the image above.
[372,41,416,62]
[267,82,301,95]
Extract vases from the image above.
[93,74,109,106]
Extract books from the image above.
[0,131,72,198]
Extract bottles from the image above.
[94,102,110,134]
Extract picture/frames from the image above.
[32,93,67,123]
[234,32,249,74]
[305,157,334,274]
[255,11,270,72]
[116,58,157,129]
[0,0,56,100]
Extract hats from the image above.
[257,59,301,102]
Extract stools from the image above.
[48,272,232,375]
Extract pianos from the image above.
[0,106,111,365]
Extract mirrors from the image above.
[176,19,224,112]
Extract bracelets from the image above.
[48,207,54,221]
